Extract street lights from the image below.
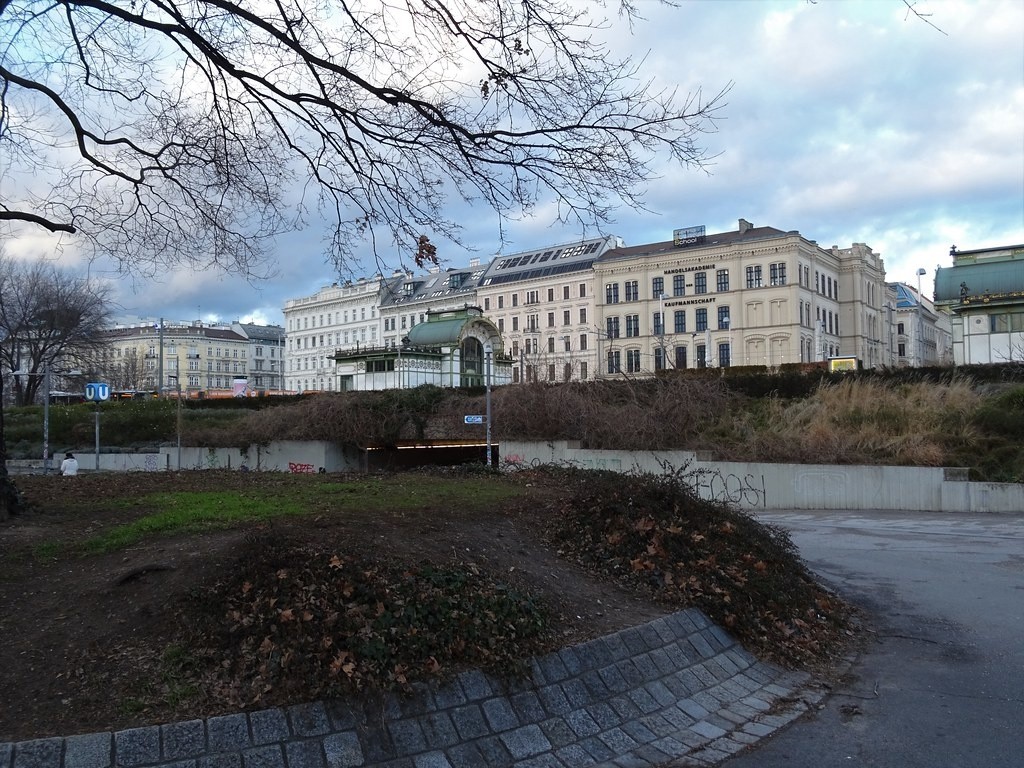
[270,330,282,391]
[723,317,732,367]
[916,268,927,367]
[659,293,671,369]
[485,344,493,466]
[9,356,50,475]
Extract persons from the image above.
[59,453,80,476]
